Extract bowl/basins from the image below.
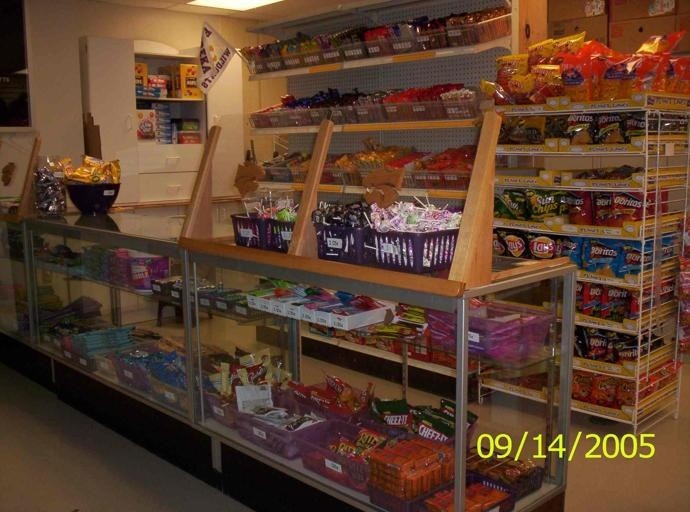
[67,184,121,214]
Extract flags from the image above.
[193,22,236,96]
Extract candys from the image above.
[32,162,68,218]
[235,190,463,234]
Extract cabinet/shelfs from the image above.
[2,217,567,511]
[502,97,687,442]
[81,35,212,205]
[244,0,548,418]
